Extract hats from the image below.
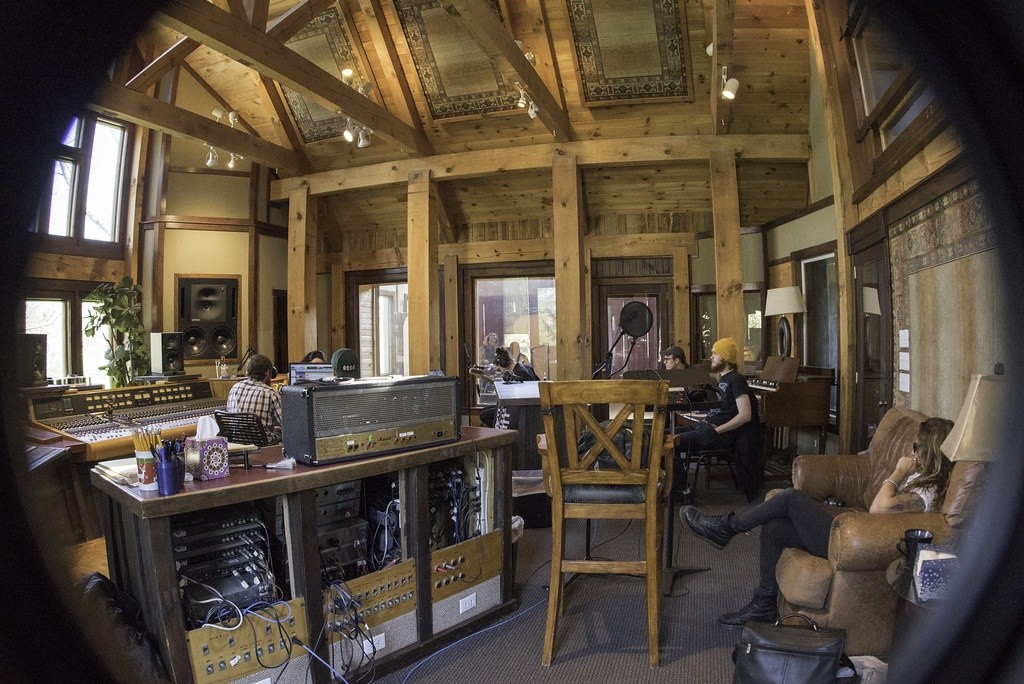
[712,337,737,363]
[659,346,686,360]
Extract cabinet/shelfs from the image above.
[90,426,518,684]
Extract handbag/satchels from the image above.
[732,611,861,684]
[582,419,650,469]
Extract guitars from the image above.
[468,365,494,385]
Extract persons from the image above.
[492,346,541,381]
[664,344,691,370]
[680,417,954,628]
[226,354,282,445]
[301,350,326,363]
[660,337,753,505]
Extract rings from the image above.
[913,457,917,461]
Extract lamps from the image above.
[862,285,880,370]
[515,40,536,120]
[237,345,279,380]
[204,109,240,171]
[705,44,739,99]
[341,70,373,149]
[939,373,1005,462]
[766,289,808,357]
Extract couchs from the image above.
[764,408,985,657]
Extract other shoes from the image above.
[672,491,692,501]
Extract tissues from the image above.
[183,416,230,481]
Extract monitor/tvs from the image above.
[288,362,332,387]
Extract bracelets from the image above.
[882,479,899,488]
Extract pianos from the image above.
[740,355,837,481]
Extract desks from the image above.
[209,374,288,399]
[886,552,947,645]
[496,382,538,471]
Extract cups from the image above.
[156,461,179,496]
[896,528,934,568]
[134,450,158,491]
[216,366,229,378]
[171,452,186,487]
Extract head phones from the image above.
[502,370,523,383]
[686,382,725,402]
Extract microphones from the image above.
[271,367,278,379]
[619,311,639,327]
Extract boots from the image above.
[717,592,779,630]
[679,504,740,549]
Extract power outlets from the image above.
[460,592,477,616]
[362,632,386,654]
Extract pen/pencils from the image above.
[131,424,187,462]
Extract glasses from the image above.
[664,357,675,361]
[913,442,921,453]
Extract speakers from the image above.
[16,334,48,387]
[150,332,183,375]
[281,376,461,466]
[176,278,237,360]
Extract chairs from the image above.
[214,409,269,449]
[685,395,757,505]
[537,380,668,669]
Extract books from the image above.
[94,458,138,485]
